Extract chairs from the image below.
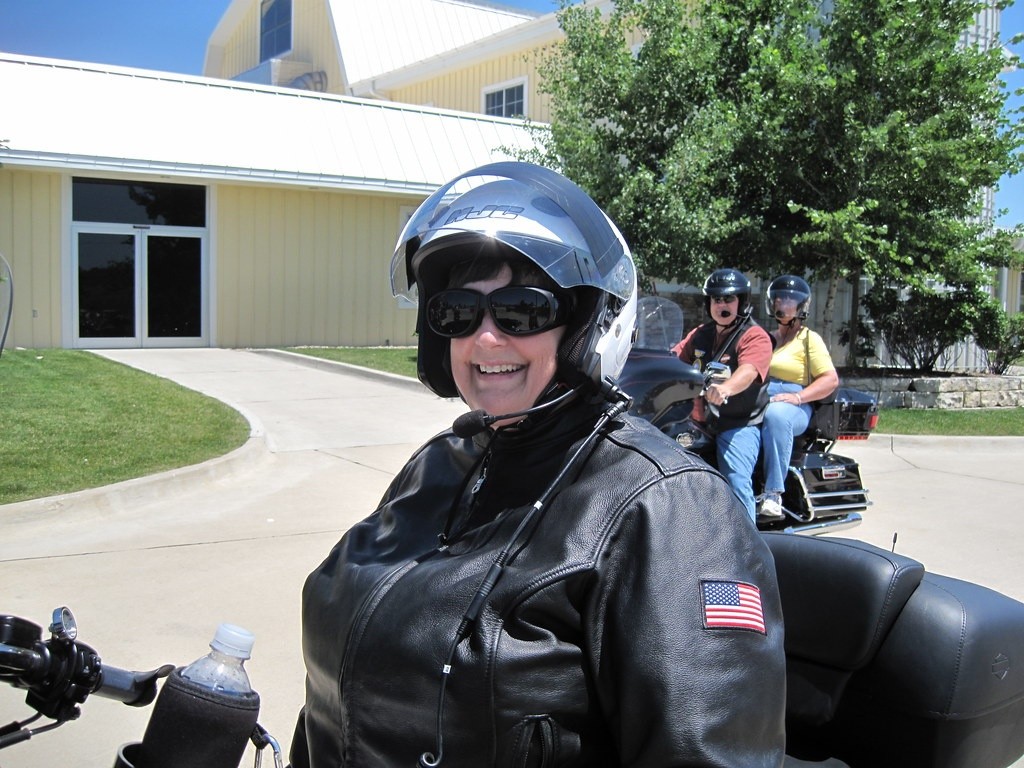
[759,530,1024,768]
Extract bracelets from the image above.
[796,393,801,406]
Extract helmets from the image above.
[701,268,752,316]
[387,162,642,405]
[765,274,813,320]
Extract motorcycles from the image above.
[618,295,879,538]
[1,536,1023,768]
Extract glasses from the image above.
[422,285,593,338]
[710,294,741,303]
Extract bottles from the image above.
[178,623,256,699]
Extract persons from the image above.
[758,275,839,517]
[289,161,786,768]
[668,269,772,524]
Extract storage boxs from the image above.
[808,387,878,441]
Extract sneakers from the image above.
[753,489,782,517]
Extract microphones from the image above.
[776,310,807,318]
[721,306,754,319]
[452,377,587,438]
